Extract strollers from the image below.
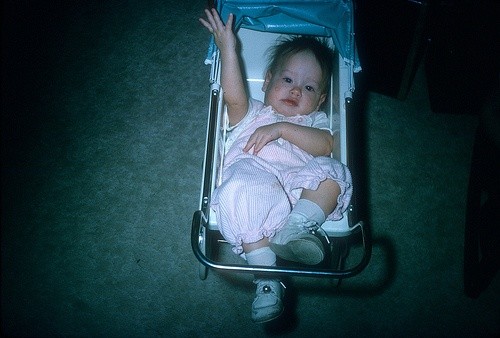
[191,0,372,286]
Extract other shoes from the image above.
[251,278,286,324]
[269,229,325,265]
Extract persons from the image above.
[198,8,353,324]
